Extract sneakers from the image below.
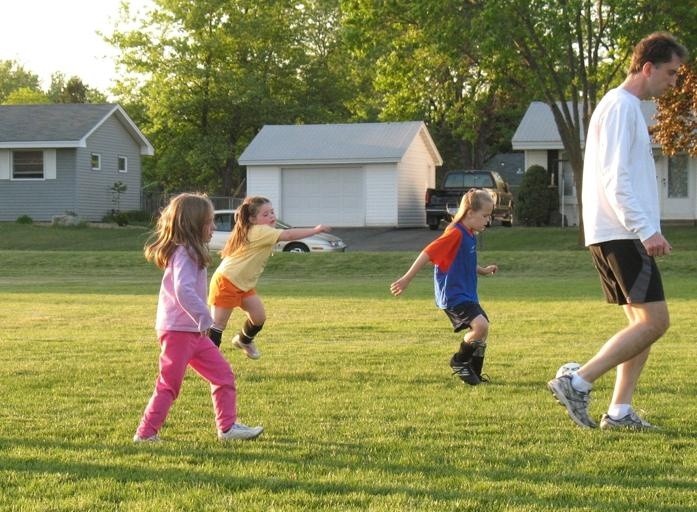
[449,353,480,386]
[132,435,162,443]
[546,373,597,428]
[231,335,260,359]
[599,413,660,430]
[218,423,263,441]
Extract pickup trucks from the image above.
[421,167,514,232]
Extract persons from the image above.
[132,192,265,443]
[205,196,330,359]
[547,30,688,434]
[390,189,498,385]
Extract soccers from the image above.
[557,363,581,378]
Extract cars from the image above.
[204,209,348,255]
